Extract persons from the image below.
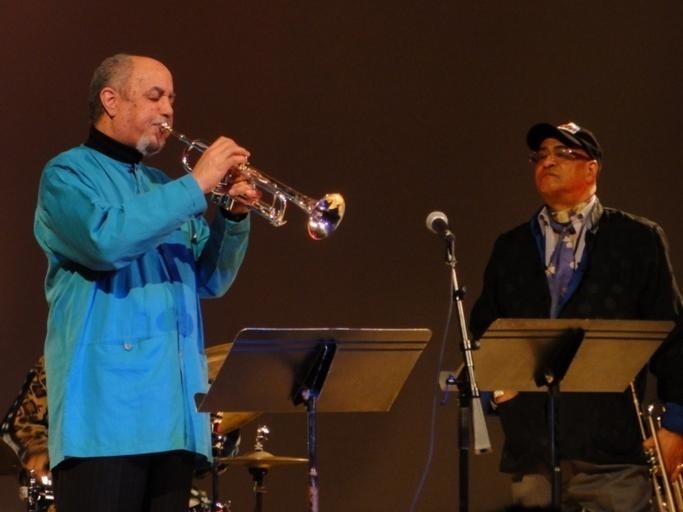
[459,118,682,511]
[30,53,261,512]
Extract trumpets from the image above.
[630,382,683,512]
[158,122,347,241]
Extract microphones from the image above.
[425,208,456,246]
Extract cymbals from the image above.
[210,454,309,468]
[205,342,264,435]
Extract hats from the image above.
[525,121,601,164]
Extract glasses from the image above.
[526,147,589,168]
